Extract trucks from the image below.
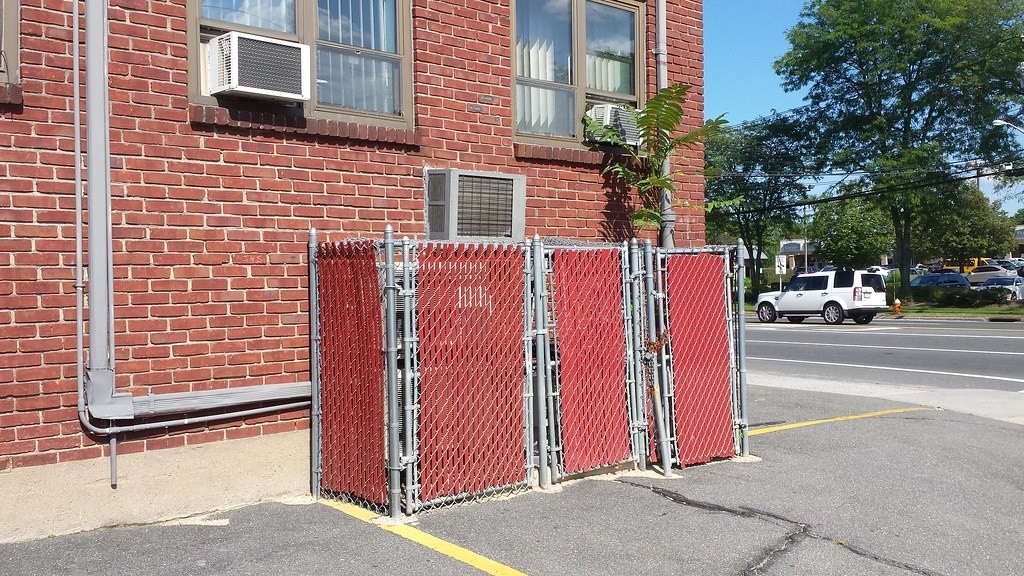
[943,257,998,273]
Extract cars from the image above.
[931,258,1024,286]
[820,267,837,271]
[974,276,1024,302]
[866,263,943,279]
[797,267,819,274]
[909,274,970,291]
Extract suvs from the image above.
[754,269,889,325]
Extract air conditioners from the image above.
[208,30,310,105]
[585,103,645,146]
[425,167,526,243]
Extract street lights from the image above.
[800,184,815,274]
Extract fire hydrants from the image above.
[893,298,902,315]
